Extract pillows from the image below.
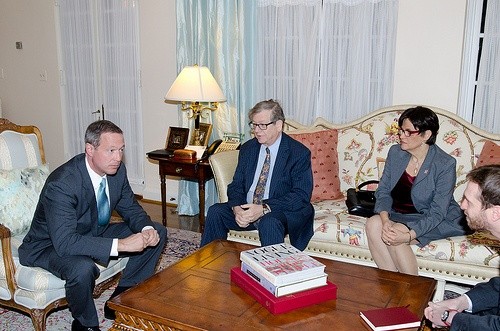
[475,141,500,168]
[0,162,52,237]
[288,130,344,202]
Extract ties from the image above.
[97,178,110,225]
[253,147,270,230]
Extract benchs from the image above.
[209,105,500,304]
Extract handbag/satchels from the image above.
[345,180,381,218]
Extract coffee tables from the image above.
[108,239,438,331]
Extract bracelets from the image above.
[406,232,411,243]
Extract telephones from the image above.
[207,139,242,156]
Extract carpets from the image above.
[0,227,203,331]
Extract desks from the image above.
[149,155,214,234]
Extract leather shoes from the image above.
[104,300,116,319]
[72,319,101,331]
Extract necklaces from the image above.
[411,157,417,174]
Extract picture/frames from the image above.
[166,127,189,152]
[190,123,213,146]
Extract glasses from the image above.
[248,121,276,130]
[398,129,421,137]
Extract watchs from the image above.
[262,203,270,215]
[441,309,459,327]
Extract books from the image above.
[240,242,328,298]
[359,304,422,331]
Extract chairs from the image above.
[0,117,129,331]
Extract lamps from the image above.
[164,64,227,146]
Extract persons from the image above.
[17,120,167,331]
[200,99,315,247]
[424,164,500,331]
[365,106,475,276]
